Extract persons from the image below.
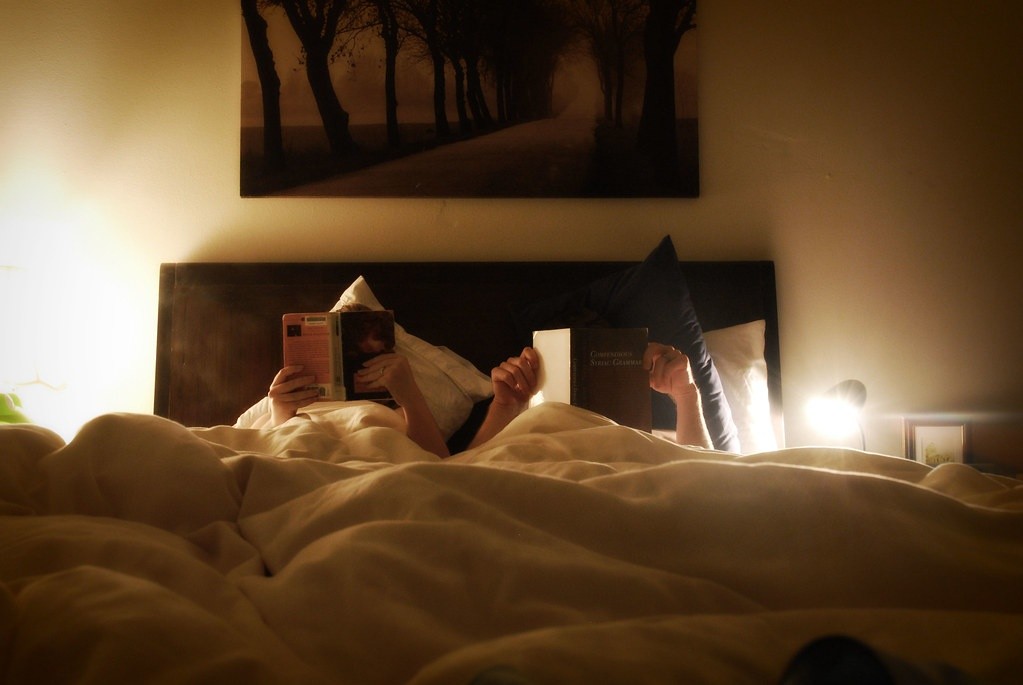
[265,353,447,465]
[452,309,720,452]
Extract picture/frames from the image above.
[902,416,967,469]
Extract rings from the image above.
[380,368,384,375]
[661,351,671,360]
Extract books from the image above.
[278,308,399,402]
[529,325,655,436]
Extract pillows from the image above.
[700,320,776,459]
[231,275,494,442]
[507,234,738,453]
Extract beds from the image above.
[0,260,1022,685]
